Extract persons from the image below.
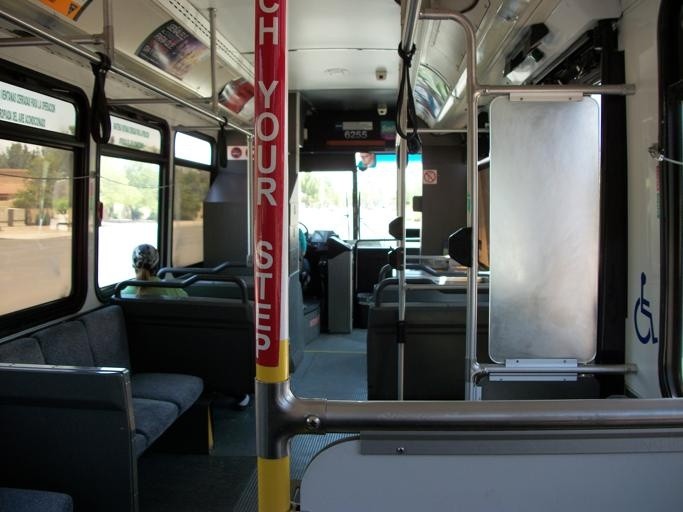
[119,244,188,296]
[356,153,376,168]
[297,227,311,288]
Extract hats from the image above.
[131,244,159,268]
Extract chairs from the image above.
[0,305,209,462]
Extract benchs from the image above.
[365,277,489,396]
[110,274,255,407]
[155,262,256,297]
[374,263,489,303]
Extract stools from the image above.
[0,489,74,511]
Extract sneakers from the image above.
[239,394,253,411]
